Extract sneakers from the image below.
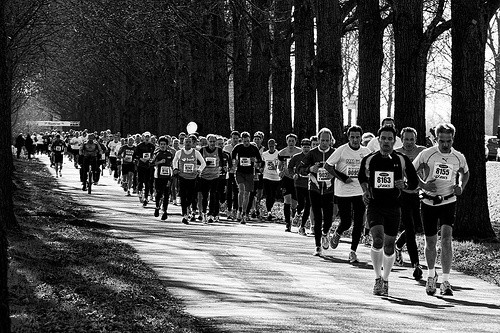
[349,252,359,263]
[313,246,322,256]
[394,240,403,266]
[413,267,423,280]
[291,212,302,226]
[439,281,454,296]
[426,270,438,295]
[330,227,342,249]
[372,276,388,296]
[322,231,329,249]
[298,227,307,236]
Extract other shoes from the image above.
[124,190,272,224]
[82,185,86,190]
[291,210,297,218]
[361,235,373,247]
[285,223,291,232]
[93,182,98,185]
[310,225,315,235]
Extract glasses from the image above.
[209,141,216,143]
[186,141,191,143]
[218,143,224,145]
[313,143,319,146]
[254,138,260,140]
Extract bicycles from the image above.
[83,155,99,194]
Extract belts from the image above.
[422,193,455,200]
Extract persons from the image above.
[324,117,470,299]
[16,126,334,235]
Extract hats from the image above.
[189,135,200,142]
[144,131,151,136]
[268,139,277,146]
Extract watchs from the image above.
[403,182,408,189]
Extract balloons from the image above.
[186,121,197,134]
